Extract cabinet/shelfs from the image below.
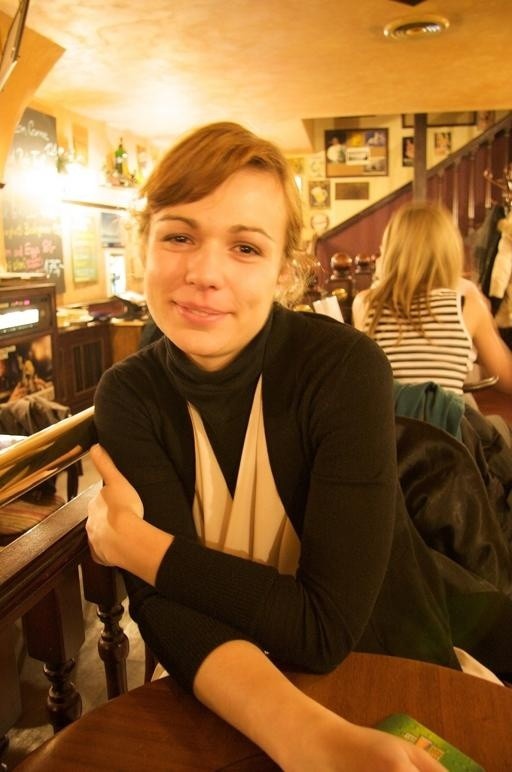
[58,323,111,417]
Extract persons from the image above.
[9,356,52,404]
[349,197,512,423]
[80,121,451,772]
[323,129,451,172]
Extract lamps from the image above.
[384,13,449,40]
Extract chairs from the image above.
[392,381,511,680]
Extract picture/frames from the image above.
[324,127,389,178]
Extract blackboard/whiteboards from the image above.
[2,106,66,308]
